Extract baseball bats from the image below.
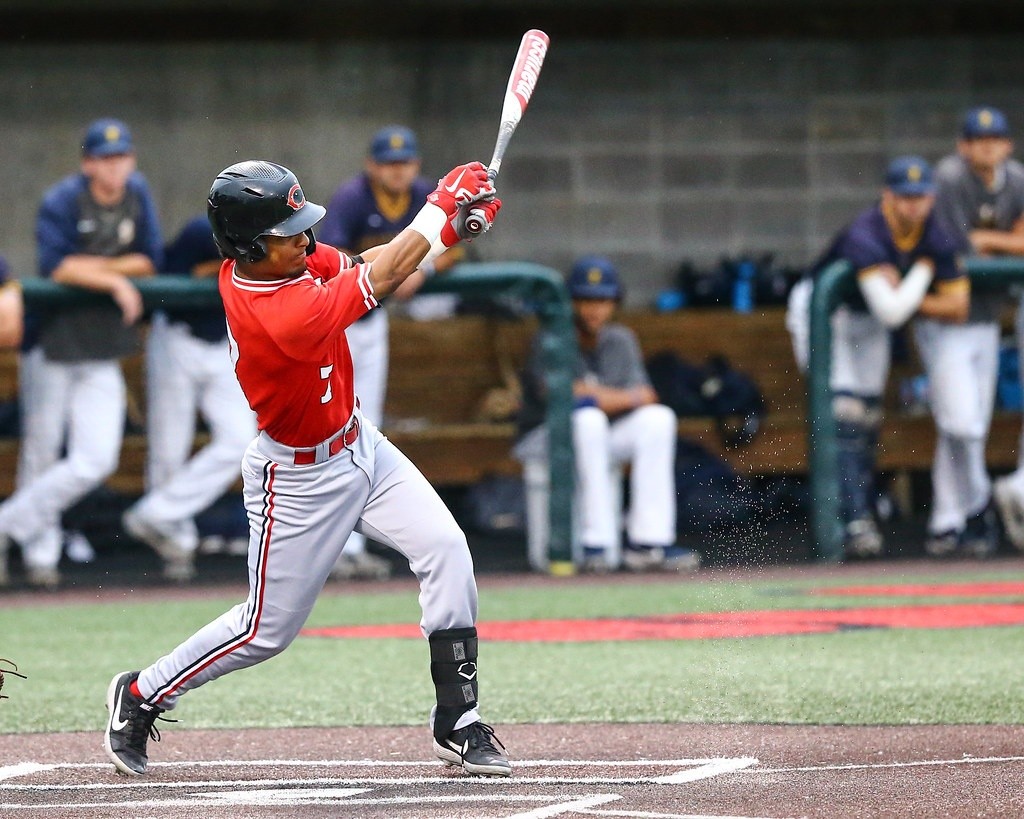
[464,27,552,232]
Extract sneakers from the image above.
[926,509,999,558]
[844,516,893,560]
[433,721,511,776]
[104,671,178,775]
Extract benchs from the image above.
[0,312,1024,535]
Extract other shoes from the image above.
[124,507,198,583]
[570,543,701,575]
[29,565,59,588]
[338,553,390,579]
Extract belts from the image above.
[295,398,359,464]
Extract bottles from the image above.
[732,262,755,314]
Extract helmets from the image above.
[207,159,325,261]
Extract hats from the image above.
[889,156,936,196]
[964,109,1013,140]
[370,123,420,163]
[81,119,135,158]
[569,256,623,300]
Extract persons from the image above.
[0,257,24,348]
[103,160,512,778]
[121,215,261,580]
[511,254,701,572]
[318,125,470,582]
[784,107,1024,562]
[0,118,166,587]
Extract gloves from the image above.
[428,163,497,216]
[443,199,501,248]
